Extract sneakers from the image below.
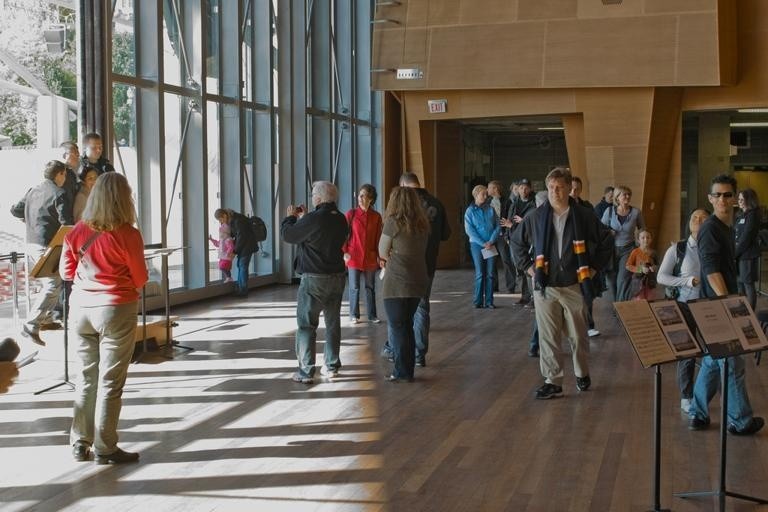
[368,319,381,323]
[351,318,358,323]
[224,277,233,284]
[689,416,710,429]
[577,375,591,391]
[587,329,600,338]
[680,399,691,412]
[319,366,338,378]
[535,384,563,399]
[231,293,249,297]
[292,373,314,383]
[727,417,764,436]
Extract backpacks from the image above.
[250,216,266,241]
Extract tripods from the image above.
[132,284,173,366]
[672,357,768,512]
[34,281,75,395]
[158,256,195,351]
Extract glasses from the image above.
[709,191,733,198]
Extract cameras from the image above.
[296,207,302,213]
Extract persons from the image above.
[377,186,431,383]
[381,172,452,367]
[215,209,259,297]
[209,223,235,283]
[509,169,615,399]
[466,177,601,356]
[0,361,19,393]
[733,189,761,313]
[658,209,712,413]
[280,181,348,383]
[594,185,660,318]
[10,134,115,346]
[58,172,148,464]
[342,184,382,324]
[688,174,763,434]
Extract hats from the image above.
[219,223,231,234]
[518,178,530,186]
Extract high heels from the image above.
[95,447,139,464]
[472,303,483,308]
[72,444,94,461]
[485,304,495,309]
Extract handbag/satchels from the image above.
[664,284,679,300]
[346,224,351,241]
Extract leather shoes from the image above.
[40,322,61,330]
[524,301,534,310]
[383,373,412,383]
[528,348,537,357]
[20,324,46,346]
[512,299,529,306]
[382,346,425,367]
